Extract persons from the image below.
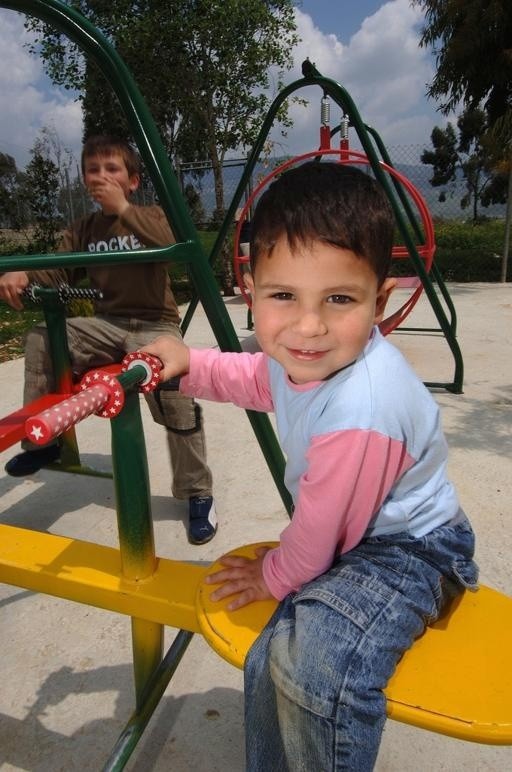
[133,161,482,772]
[0,136,217,545]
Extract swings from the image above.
[233,95,436,337]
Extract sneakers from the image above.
[189,495,218,545]
[4,446,61,477]
